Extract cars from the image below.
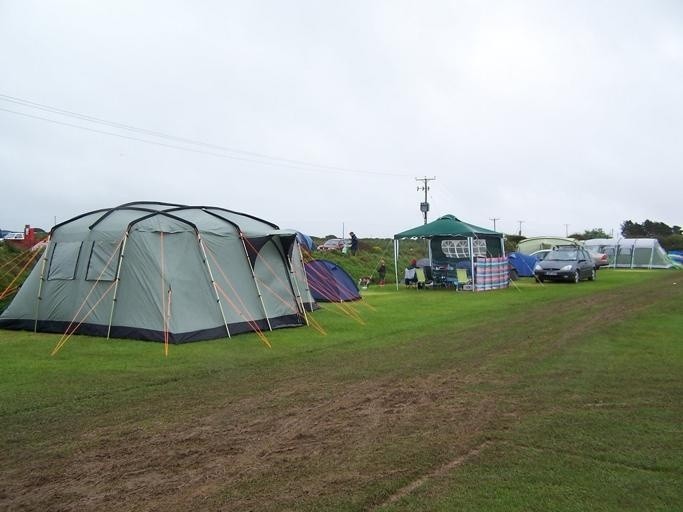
[316,239,344,251]
[528,249,551,261]
[533,244,595,284]
[0,233,24,241]
[588,250,608,270]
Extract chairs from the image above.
[456,268,470,291]
[405,265,435,291]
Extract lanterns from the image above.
[507,252,539,278]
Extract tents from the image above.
[581,237,674,269]
[515,237,585,257]
[0,199,320,346]
[301,259,362,305]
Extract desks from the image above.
[433,269,455,288]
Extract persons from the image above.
[405,259,417,285]
[377,264,386,285]
[349,232,358,256]
